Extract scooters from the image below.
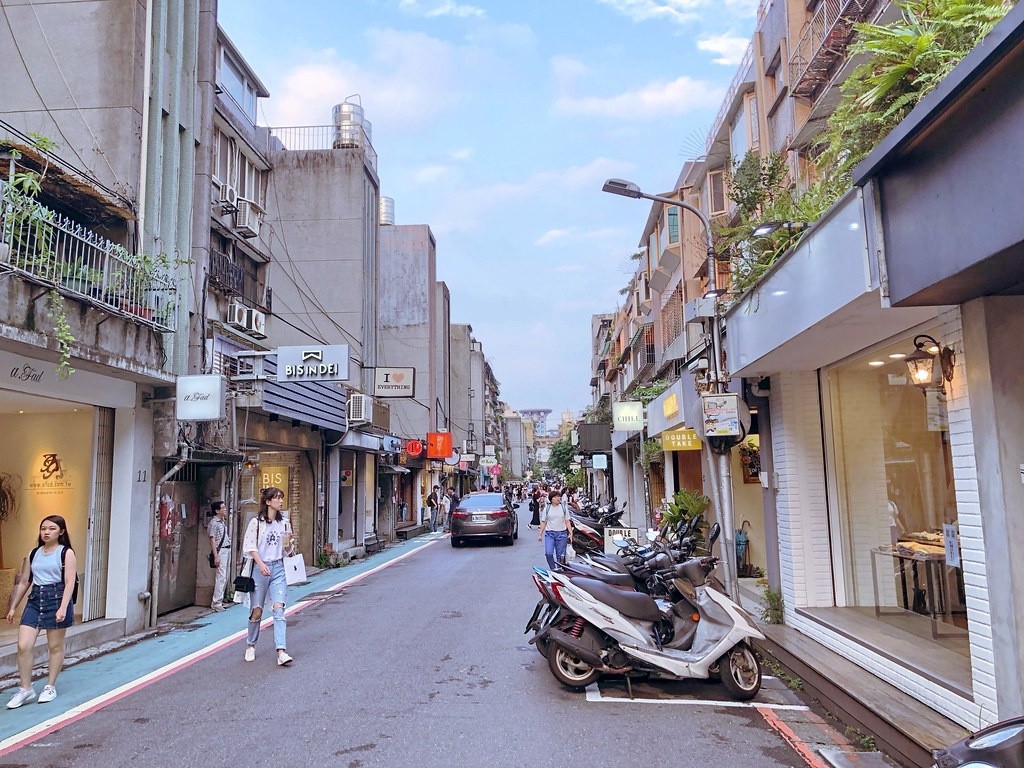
[524,515,732,659]
[529,523,769,700]
[928,716,1024,768]
[568,493,627,553]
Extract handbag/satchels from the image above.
[209,553,216,568]
[565,543,576,564]
[283,549,307,586]
[233,576,255,593]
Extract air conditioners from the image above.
[227,303,266,339]
[350,394,372,424]
[220,184,260,238]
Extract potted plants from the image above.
[0,132,195,382]
[0,472,23,618]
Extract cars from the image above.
[451,492,520,547]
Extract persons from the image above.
[538,491,573,570]
[429,485,442,534]
[207,501,231,612]
[527,484,580,530]
[442,486,460,533]
[6,515,77,709]
[242,487,295,666]
[501,483,530,503]
[470,485,501,493]
[888,500,905,545]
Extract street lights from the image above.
[603,178,740,611]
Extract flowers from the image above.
[737,437,761,475]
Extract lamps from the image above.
[753,221,816,238]
[311,424,319,432]
[701,288,744,300]
[904,333,955,398]
[292,419,300,428]
[269,413,278,423]
[244,460,253,469]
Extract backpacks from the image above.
[529,500,536,511]
[426,492,436,507]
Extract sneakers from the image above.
[6,688,37,708]
[211,601,226,612]
[277,653,293,666]
[538,527,540,531]
[245,647,255,661]
[38,685,57,702]
[527,524,532,531]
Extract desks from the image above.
[871,536,967,639]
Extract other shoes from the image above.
[429,530,450,535]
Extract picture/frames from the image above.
[743,455,762,483]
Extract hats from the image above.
[434,485,441,489]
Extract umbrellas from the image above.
[735,520,751,570]
